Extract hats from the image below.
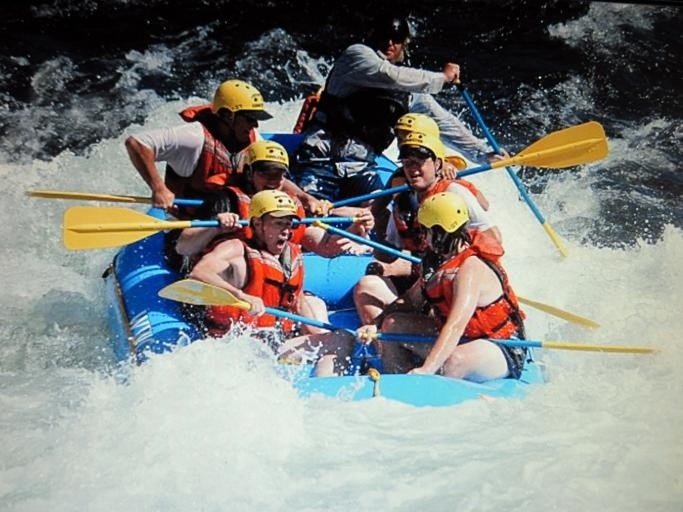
[368,13,409,41]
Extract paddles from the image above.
[157,279,363,338]
[372,334,660,352]
[324,121,609,209]
[25,190,203,205]
[63,206,363,249]
[313,220,599,328]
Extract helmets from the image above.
[211,80,301,228]
[394,112,469,234]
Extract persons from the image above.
[370,113,488,215]
[378,191,527,380]
[173,139,373,258]
[125,79,326,217]
[302,12,510,196]
[352,132,503,323]
[188,190,352,380]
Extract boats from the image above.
[102,134,547,407]
